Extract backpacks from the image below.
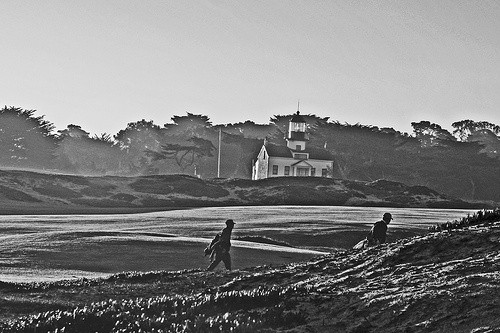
[203,234,221,257]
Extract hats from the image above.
[225,218,235,225]
[382,212,394,220]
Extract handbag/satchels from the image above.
[353,237,370,250]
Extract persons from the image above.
[205,219,236,271]
[366,212,393,248]
